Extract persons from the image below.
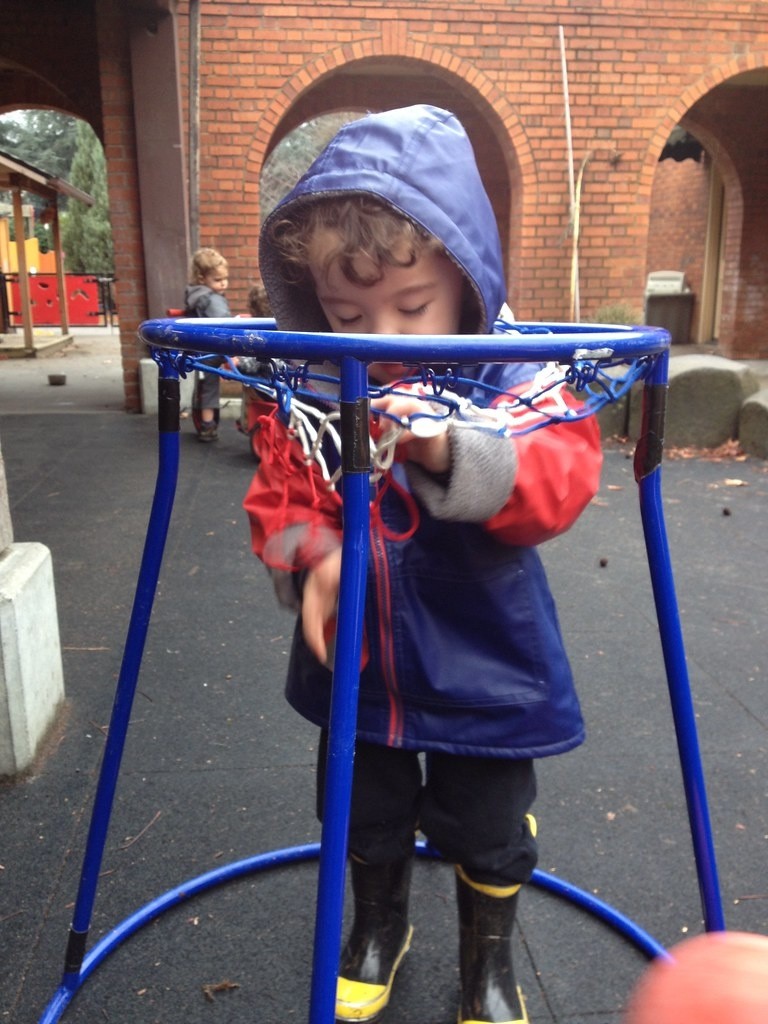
[244,107,601,1024]
[185,249,231,439]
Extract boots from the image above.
[451,813,537,1023]
[333,817,424,1022]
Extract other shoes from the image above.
[197,420,220,442]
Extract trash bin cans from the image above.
[645,270,697,344]
